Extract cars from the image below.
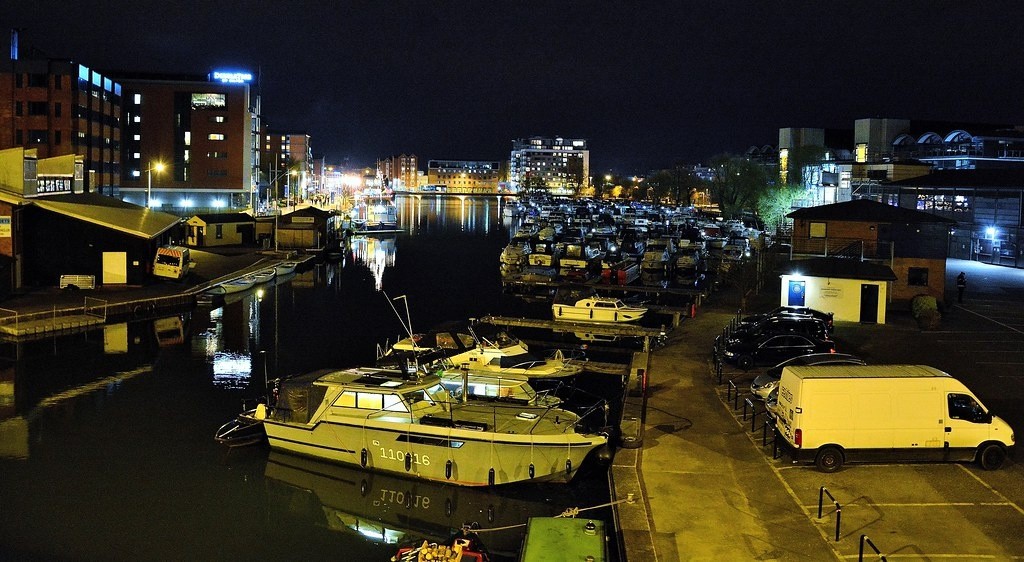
[724,305,871,413]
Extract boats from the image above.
[262,446,592,562]
[235,365,613,487]
[356,186,766,428]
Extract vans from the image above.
[155,316,186,350]
[773,364,1017,475]
[153,245,191,285]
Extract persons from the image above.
[916,272,928,286]
[957,272,968,306]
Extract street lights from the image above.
[147,162,166,210]
